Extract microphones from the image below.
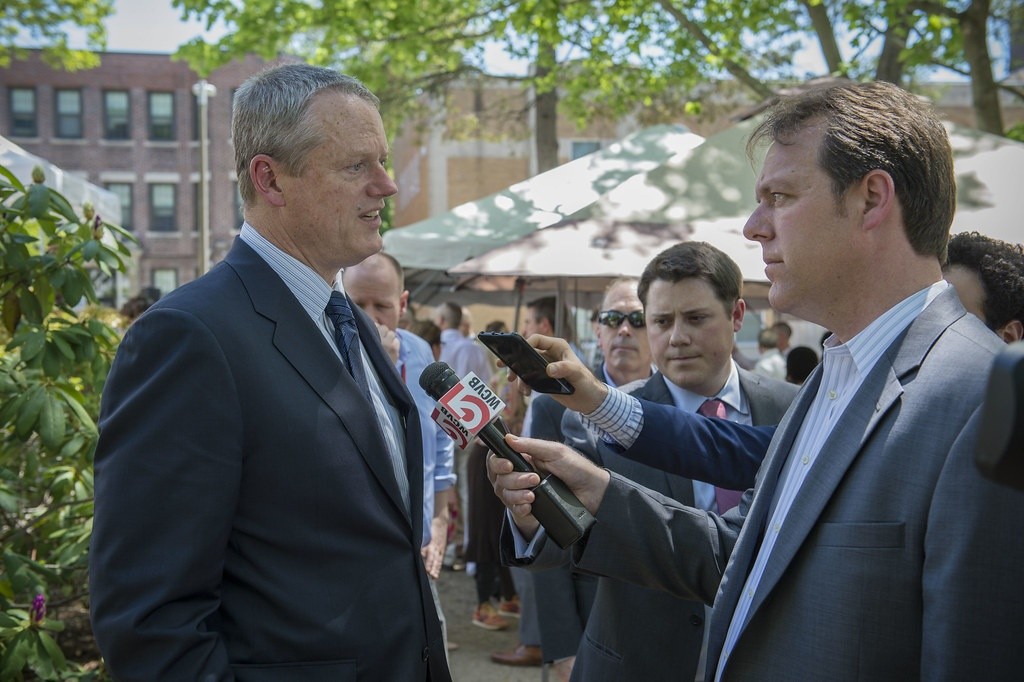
[419,361,598,550]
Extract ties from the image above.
[697,401,744,515]
[326,297,394,475]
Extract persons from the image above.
[493,230,1024,491]
[90,65,452,682]
[486,243,801,682]
[488,80,1024,681]
[338,250,457,673]
[399,279,833,682]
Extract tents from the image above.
[374,115,1024,354]
[1,135,128,321]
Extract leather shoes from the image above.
[492,645,543,667]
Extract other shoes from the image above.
[453,558,466,570]
[472,603,509,629]
[498,599,522,618]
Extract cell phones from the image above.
[478,331,574,394]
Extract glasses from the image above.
[599,310,647,328]
